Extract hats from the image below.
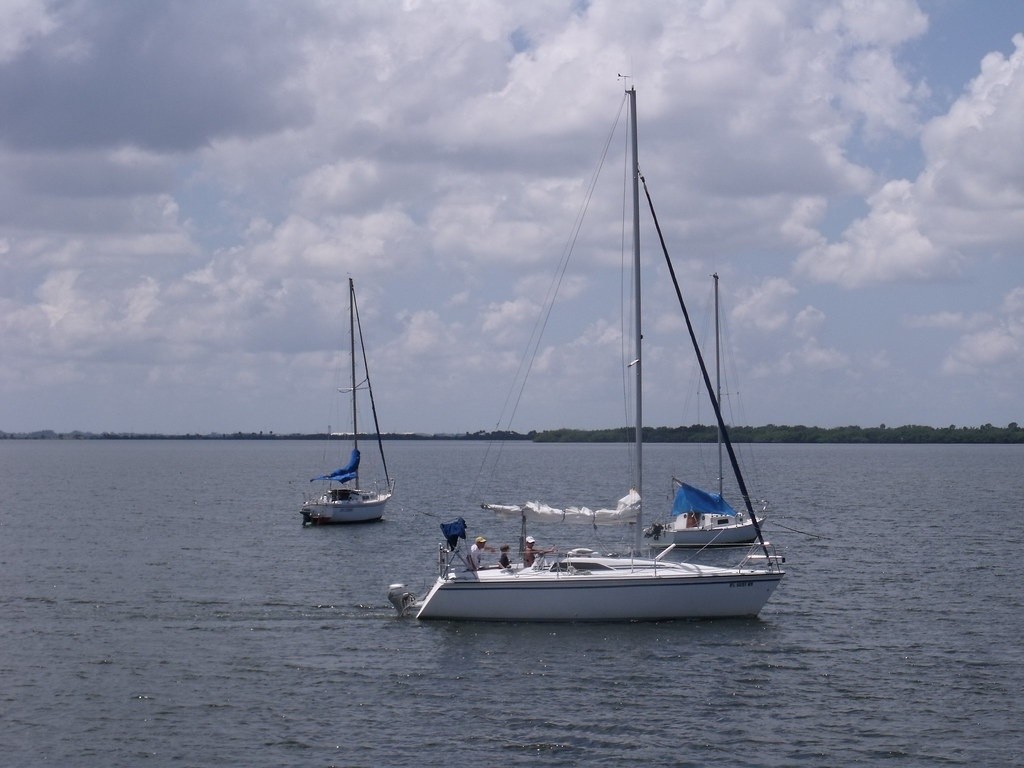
[475,537,487,542]
[500,545,509,551]
[526,536,536,543]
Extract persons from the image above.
[524,536,555,567]
[499,545,512,569]
[466,537,496,570]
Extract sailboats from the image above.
[645,273,767,548]
[387,72,788,624]
[298,277,396,525]
